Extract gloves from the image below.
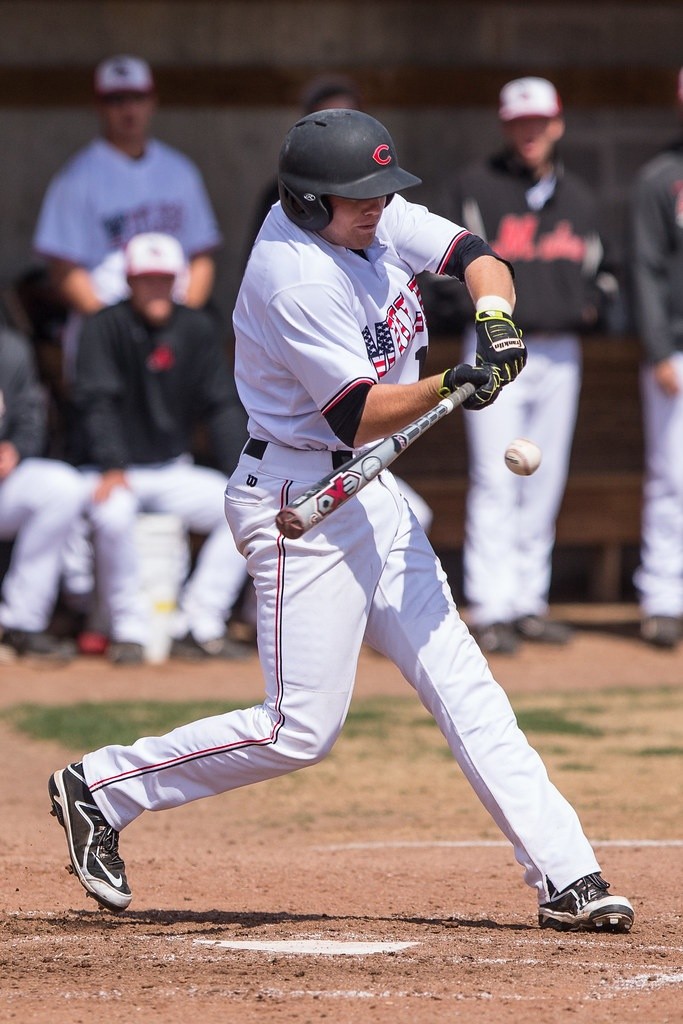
[473,309,529,385]
[438,362,505,410]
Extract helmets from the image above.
[276,108,423,235]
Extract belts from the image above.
[243,438,352,471]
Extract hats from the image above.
[95,52,157,106]
[496,74,561,126]
[123,229,188,281]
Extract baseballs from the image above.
[502,438,545,478]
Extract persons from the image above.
[462,78,620,653]
[629,72,683,648]
[48,109,633,931]
[0,313,82,662]
[251,87,359,248]
[34,52,226,312]
[63,230,246,663]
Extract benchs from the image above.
[403,476,646,604]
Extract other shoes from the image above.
[520,610,573,644]
[475,621,517,656]
[640,612,678,650]
[206,637,253,659]
[4,628,75,662]
[106,640,148,669]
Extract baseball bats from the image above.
[274,383,476,544]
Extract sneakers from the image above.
[537,873,635,931]
[46,759,136,914]
